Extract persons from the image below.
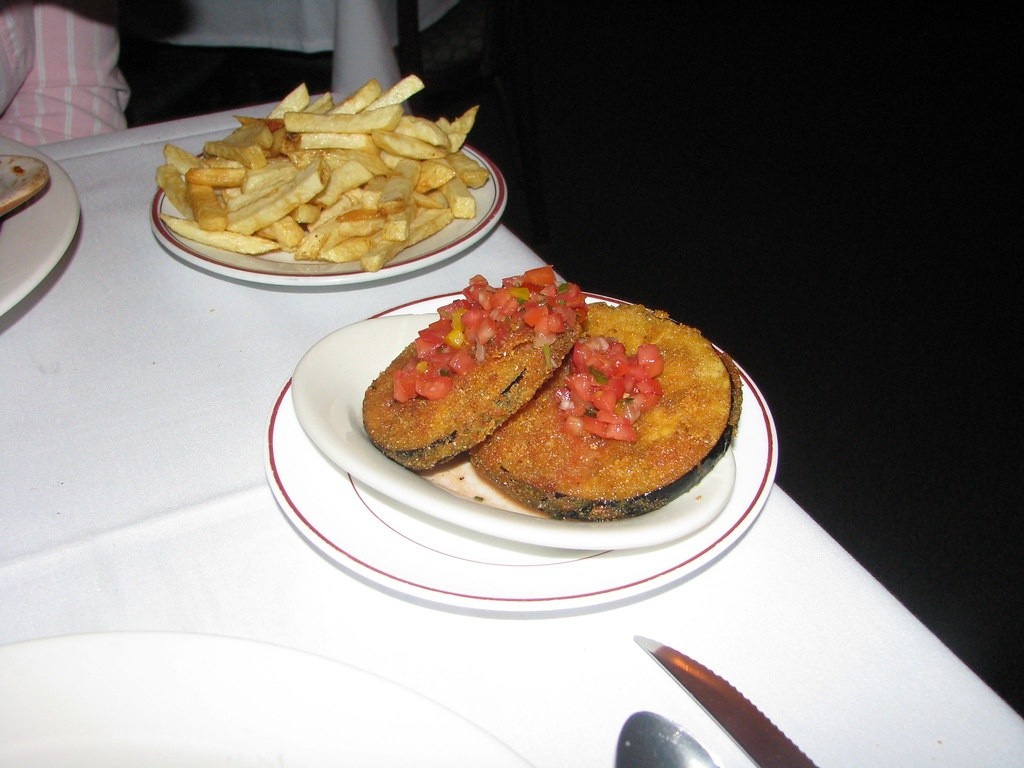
[0,0,124,146]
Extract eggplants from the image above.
[361,298,578,473]
[468,300,740,522]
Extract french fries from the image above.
[154,73,491,273]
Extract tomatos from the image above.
[392,262,666,444]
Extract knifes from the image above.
[633,635,819,768]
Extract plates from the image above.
[0,134,80,320]
[150,140,508,286]
[1,631,542,767]
[291,313,736,550]
[263,287,779,610]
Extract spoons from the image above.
[615,712,716,768]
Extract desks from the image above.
[0,95,1024,768]
